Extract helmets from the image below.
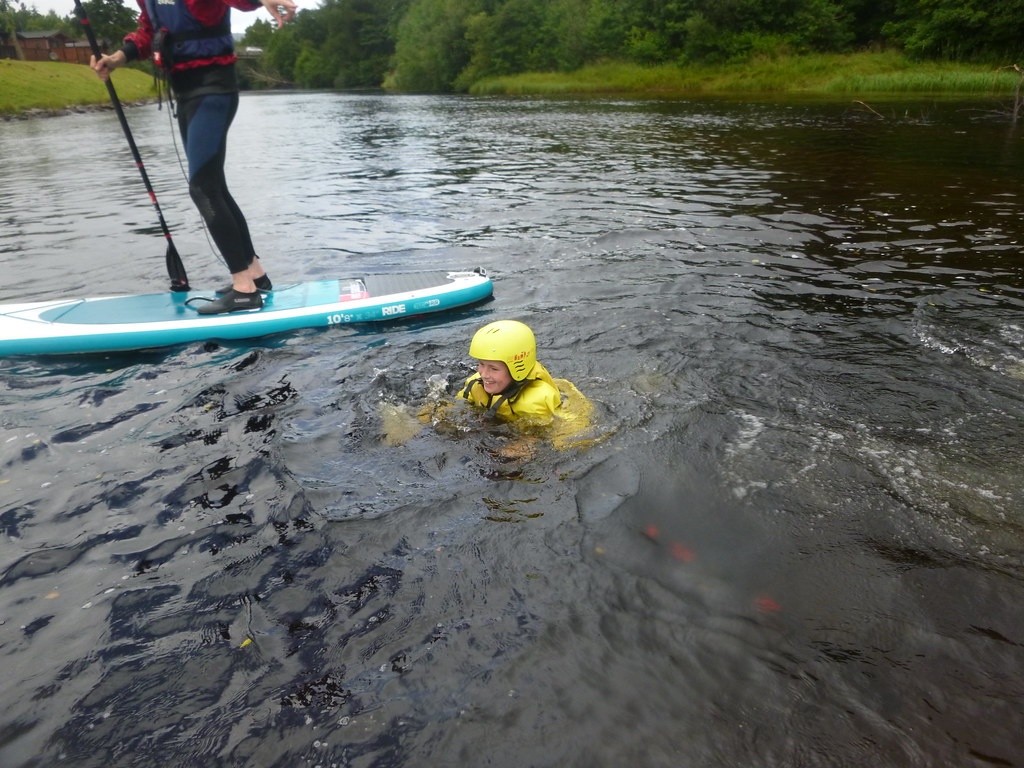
[469,320,536,381]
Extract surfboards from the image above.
[3,268,496,358]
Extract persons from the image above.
[379,321,564,480]
[89,0,299,315]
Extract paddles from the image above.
[75,0,191,293]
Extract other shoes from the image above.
[198,285,263,314]
[216,273,273,295]
